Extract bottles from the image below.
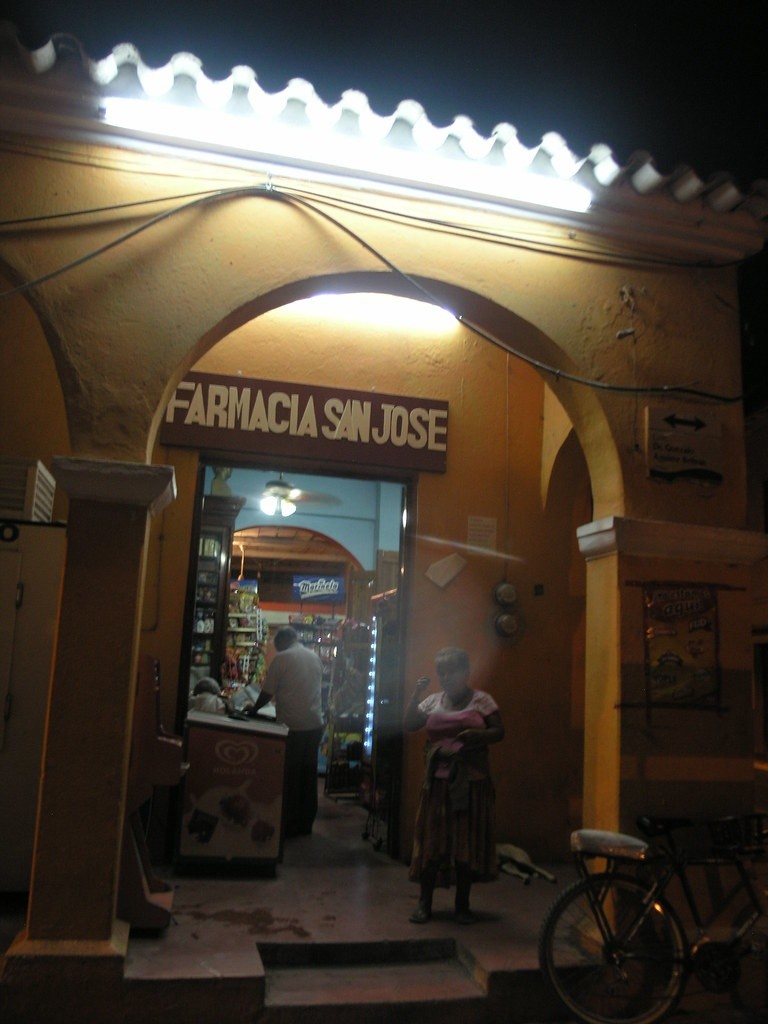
[190,606,217,667]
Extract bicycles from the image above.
[536,760,768,1024]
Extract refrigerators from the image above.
[174,709,290,877]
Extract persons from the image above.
[242,628,326,837]
[402,643,505,926]
[193,677,232,715]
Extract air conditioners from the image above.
[0,456,55,523]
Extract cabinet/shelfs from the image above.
[290,615,371,801]
[189,492,266,689]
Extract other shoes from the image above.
[408,909,433,923]
[454,910,475,924]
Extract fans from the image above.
[241,471,342,501]
